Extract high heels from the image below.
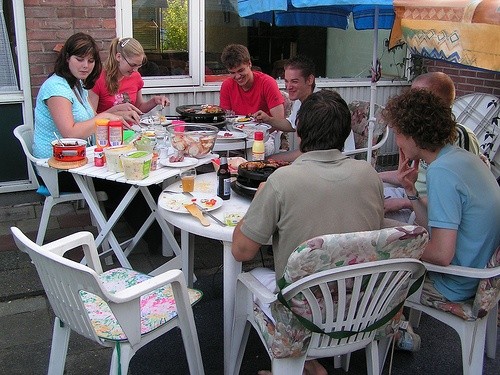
[316,357,347,374]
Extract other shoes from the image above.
[393,314,422,352]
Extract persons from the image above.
[254,56,355,164]
[377,72,500,351]
[231,89,384,375]
[33,33,140,223]
[88,37,170,131]
[220,45,284,134]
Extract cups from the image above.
[133,139,157,151]
[180,167,196,193]
[172,121,185,137]
[226,110,235,120]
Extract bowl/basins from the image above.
[102,145,134,172]
[120,151,154,180]
[51,139,88,162]
[165,123,219,159]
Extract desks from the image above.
[159,171,272,375]
[453,94,500,180]
[210,131,269,156]
[36,144,219,282]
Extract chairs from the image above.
[279,90,293,152]
[10,226,205,375]
[14,124,113,266]
[378,248,500,374]
[226,257,425,375]
[340,101,388,165]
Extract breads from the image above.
[173,134,215,158]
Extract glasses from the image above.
[121,55,143,69]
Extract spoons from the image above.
[164,190,194,197]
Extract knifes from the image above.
[193,202,226,227]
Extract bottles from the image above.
[252,132,265,161]
[216,153,231,200]
[151,151,160,170]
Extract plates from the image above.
[142,119,175,126]
[160,157,199,167]
[222,115,272,131]
[158,189,224,213]
[176,104,225,117]
[238,160,292,180]
[141,131,170,139]
[217,130,248,140]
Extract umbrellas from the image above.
[238,0,396,165]
